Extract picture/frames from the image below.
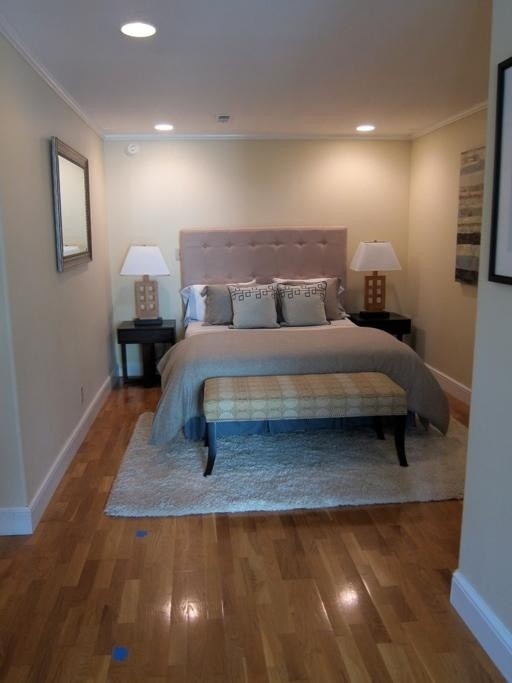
[486,53,512,285]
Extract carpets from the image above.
[103,411,467,517]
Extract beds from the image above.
[178,224,416,441]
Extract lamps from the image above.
[119,243,172,325]
[349,238,402,317]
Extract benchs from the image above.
[203,370,408,476]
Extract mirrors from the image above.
[49,133,94,272]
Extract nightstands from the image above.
[117,317,177,386]
[349,311,411,341]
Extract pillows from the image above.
[181,269,351,328]
